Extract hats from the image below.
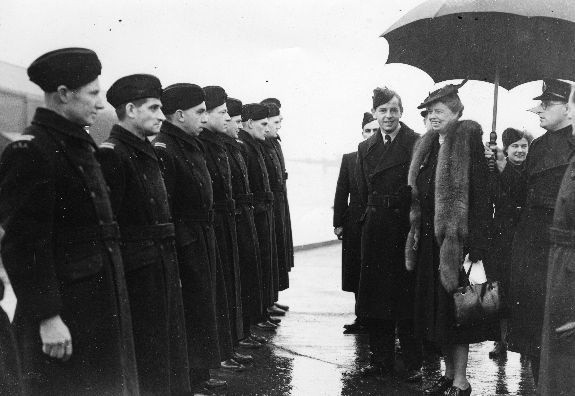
[106,73,162,106]
[226,97,242,116]
[266,103,280,117]
[372,85,395,108]
[260,98,281,108]
[242,103,269,121]
[27,47,102,92]
[161,83,206,115]
[362,111,377,129]
[202,86,227,109]
[502,128,523,146]
[533,77,571,101]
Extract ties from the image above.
[384,135,392,149]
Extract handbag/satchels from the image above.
[452,261,505,345]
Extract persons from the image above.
[258,102,294,316]
[489,128,532,362]
[333,111,379,333]
[535,89,574,395]
[152,82,229,396]
[201,86,254,374]
[357,88,422,382]
[262,98,283,122]
[1,48,140,395]
[227,95,269,349]
[238,105,281,332]
[96,73,189,396]
[483,77,573,386]
[421,110,432,131]
[398,85,489,396]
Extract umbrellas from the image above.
[380,0,575,172]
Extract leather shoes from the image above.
[268,308,285,316]
[425,376,453,395]
[250,334,267,343]
[250,320,278,331]
[441,383,471,396]
[343,317,364,330]
[274,303,289,311]
[233,353,253,363]
[238,336,262,348]
[197,379,228,389]
[361,363,391,376]
[220,358,246,371]
[193,387,216,396]
[269,318,281,323]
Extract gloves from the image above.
[469,249,488,263]
[398,184,412,208]
